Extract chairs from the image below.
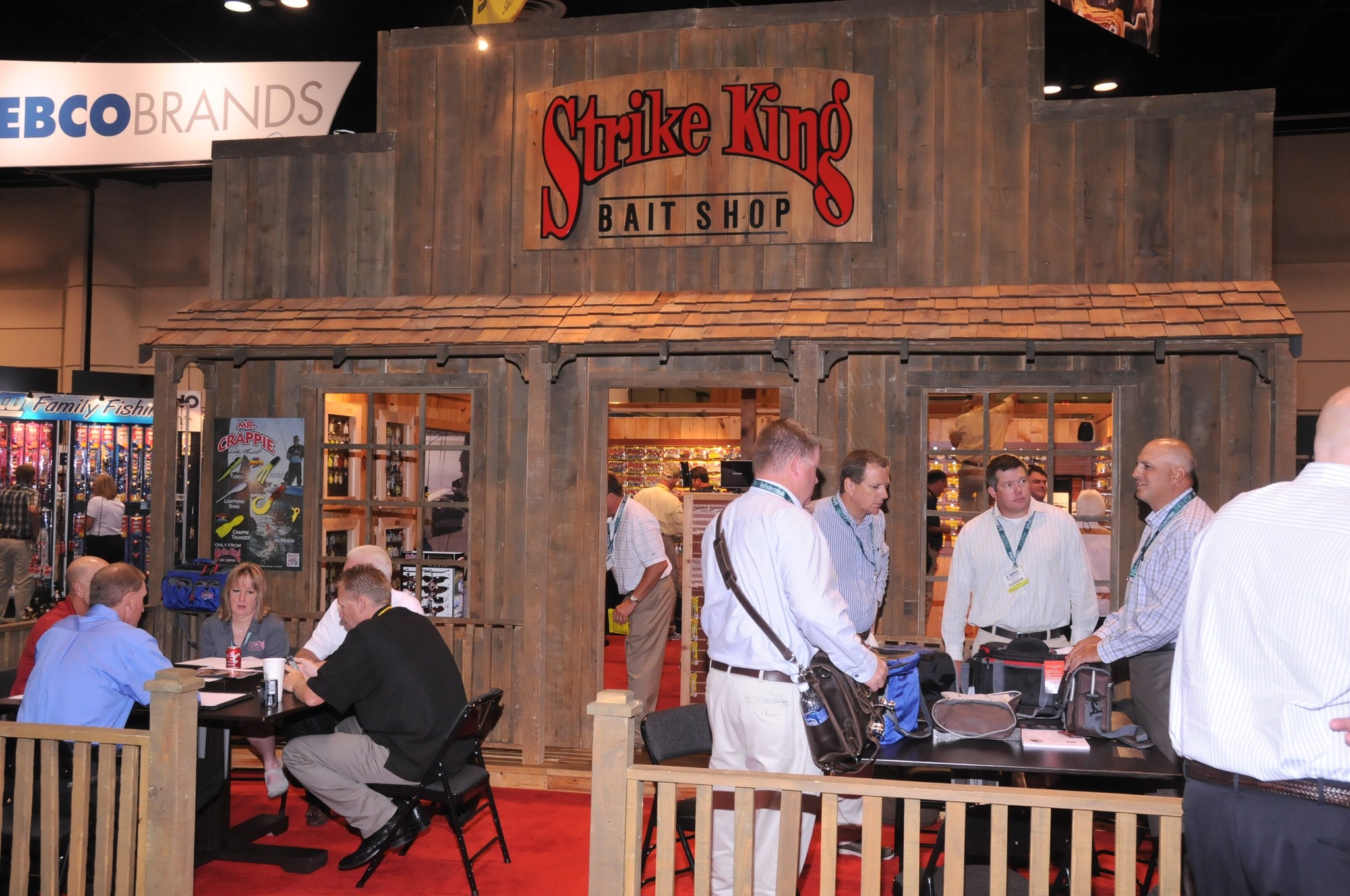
[892,767,1072,896]
[0,740,97,886]
[640,703,712,885]
[355,688,510,895]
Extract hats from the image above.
[662,465,680,478]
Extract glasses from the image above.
[399,575,449,613]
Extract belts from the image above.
[979,625,1070,641]
[1149,642,1176,652]
[857,629,872,641]
[1183,761,1350,808]
[964,460,983,467]
[626,590,634,596]
[709,660,793,682]
[930,544,939,551]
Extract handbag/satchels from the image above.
[163,560,232,613]
[1059,658,1113,737]
[868,644,957,745]
[963,636,1069,721]
[799,653,886,773]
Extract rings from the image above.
[619,617,623,620]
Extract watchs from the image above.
[630,595,639,604]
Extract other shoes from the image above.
[264,758,289,798]
[836,841,895,861]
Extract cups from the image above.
[263,658,287,702]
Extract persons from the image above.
[699,418,888,895]
[606,471,677,752]
[802,449,894,859]
[689,466,720,493]
[1026,464,1047,502]
[84,474,125,565]
[925,469,951,631]
[631,465,684,640]
[1063,438,1215,837]
[0,465,42,624]
[1168,385,1350,896]
[1072,490,1112,633]
[198,562,290,798]
[941,452,1099,677]
[948,393,1020,524]
[8,556,220,814]
[283,544,468,870]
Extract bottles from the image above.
[55,590,61,605]
[799,682,830,726]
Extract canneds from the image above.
[259,679,278,708]
[225,646,242,669]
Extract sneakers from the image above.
[305,793,331,826]
[667,631,682,641]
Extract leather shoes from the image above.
[400,799,432,833]
[339,812,414,871]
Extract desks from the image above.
[0,668,328,873]
[857,727,1184,875]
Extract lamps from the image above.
[451,4,487,51]
[99,394,105,401]
[26,391,46,398]
